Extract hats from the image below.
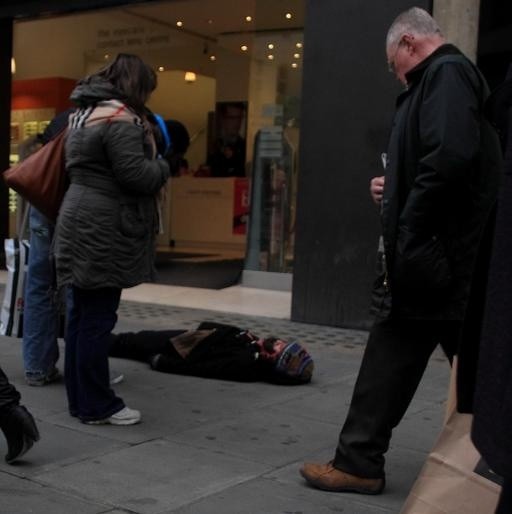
[275,341,314,383]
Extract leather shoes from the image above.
[300,462,386,495]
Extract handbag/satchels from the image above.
[2,121,68,221]
[0,238,33,339]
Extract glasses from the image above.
[387,43,398,74]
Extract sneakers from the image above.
[110,370,124,384]
[83,406,141,424]
[27,370,64,386]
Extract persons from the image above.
[108,322,313,386]
[0,369,40,464]
[50,52,170,426]
[23,110,75,386]
[300,6,511,496]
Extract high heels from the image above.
[0,404,41,462]
